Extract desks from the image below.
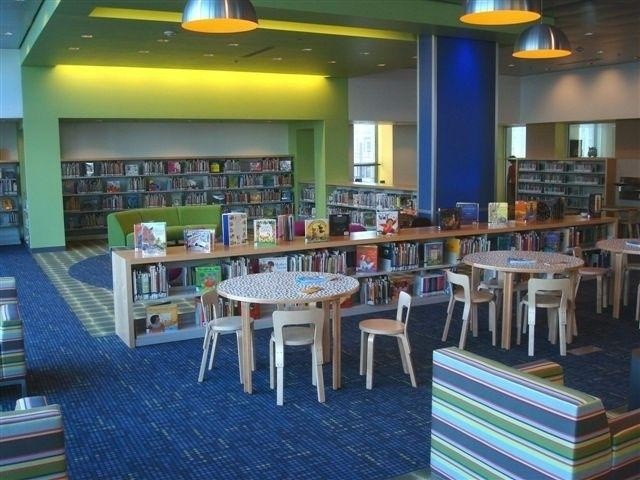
[597,237,640,321]
[461,251,585,351]
[215,271,357,391]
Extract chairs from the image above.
[573,245,609,317]
[357,291,418,391]
[441,266,496,352]
[197,287,254,384]
[267,308,328,407]
[515,274,576,358]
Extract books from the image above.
[457,202,479,224]
[222,213,247,245]
[515,173,605,186]
[329,187,416,210]
[423,241,444,267]
[221,201,291,218]
[513,188,603,207]
[258,249,346,274]
[519,159,603,172]
[145,302,179,332]
[297,202,316,217]
[195,297,259,326]
[60,159,293,176]
[580,250,610,269]
[61,189,293,209]
[141,222,166,258]
[305,218,330,243]
[513,224,610,252]
[2,212,19,224]
[488,203,508,223]
[183,229,215,253]
[299,184,315,200]
[381,242,421,272]
[63,176,292,193]
[328,205,416,236]
[279,214,295,241]
[438,208,461,231]
[223,258,252,278]
[625,239,640,248]
[195,264,221,292]
[1,180,20,196]
[446,234,514,264]
[63,213,106,230]
[254,218,277,247]
[359,274,448,306]
[356,244,378,272]
[134,223,142,252]
[132,262,169,301]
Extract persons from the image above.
[505,156,517,189]
[148,314,166,332]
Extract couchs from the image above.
[0,271,28,394]
[0,393,70,480]
[106,204,225,249]
[430,346,640,480]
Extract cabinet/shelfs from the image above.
[0,161,23,246]
[61,154,299,240]
[112,216,619,347]
[324,182,417,229]
[506,156,618,214]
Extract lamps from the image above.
[180,0,261,37]
[459,0,543,26]
[511,23,574,61]
